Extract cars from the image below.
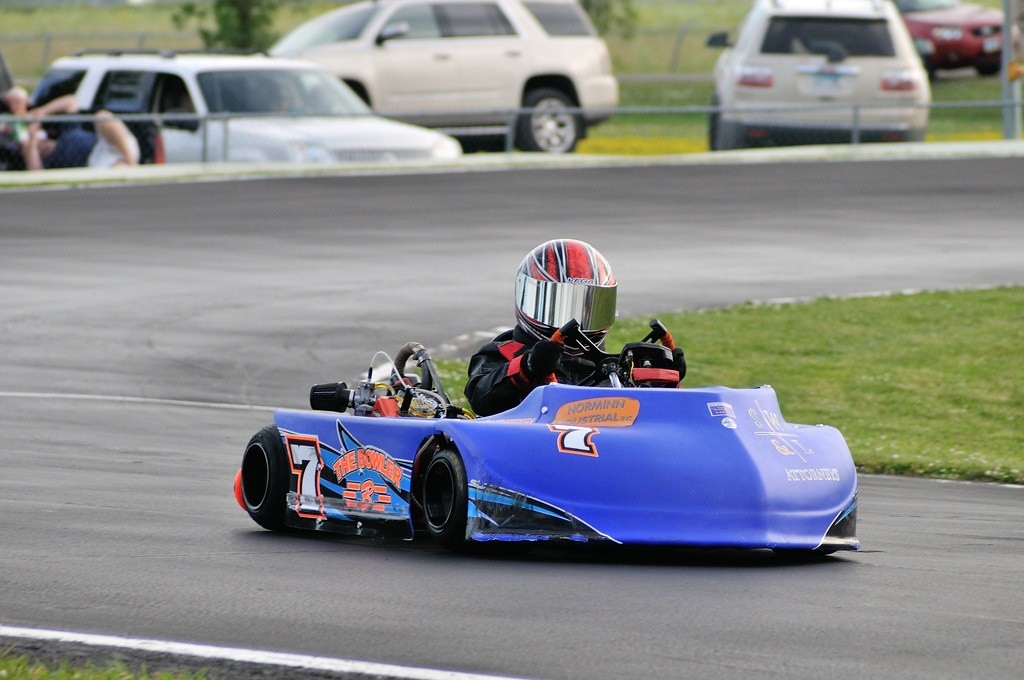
[893,0,1020,80]
[265,0,622,151]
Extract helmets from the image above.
[514,238,619,357]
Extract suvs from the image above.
[29,49,467,164]
[705,1,932,151]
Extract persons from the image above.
[0,52,140,170]
[464,239,687,416]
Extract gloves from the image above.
[521,340,565,384]
[671,347,686,382]
